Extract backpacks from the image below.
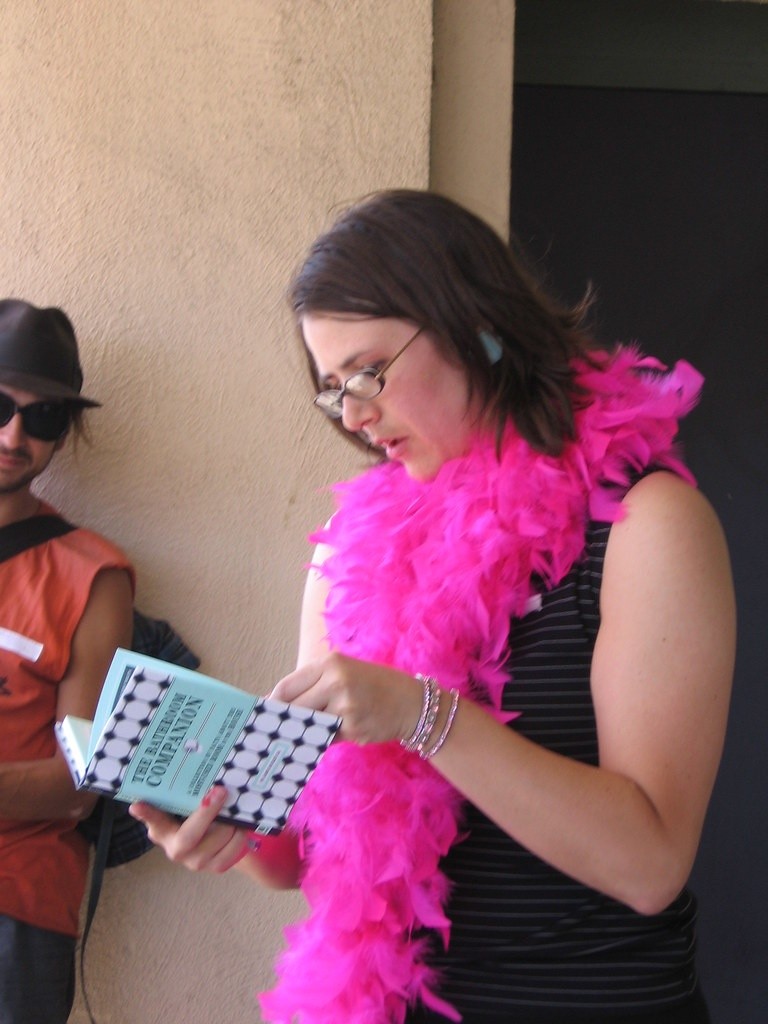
[77,606,201,869]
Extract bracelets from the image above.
[399,673,461,759]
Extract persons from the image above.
[0,297,137,1024]
[123,188,732,1022]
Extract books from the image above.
[51,647,344,837]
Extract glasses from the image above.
[312,325,429,418]
[0,390,71,441]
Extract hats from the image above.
[0,299,103,407]
